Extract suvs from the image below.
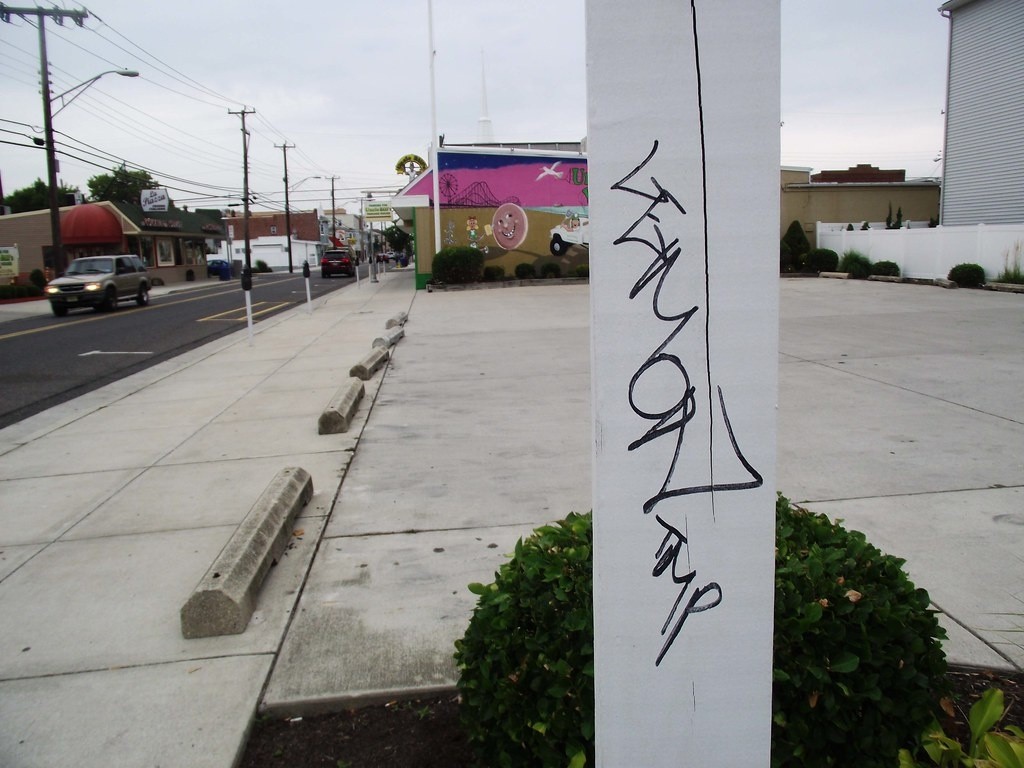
[320,250,356,278]
[47,254,153,316]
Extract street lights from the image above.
[0,3,140,277]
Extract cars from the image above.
[387,252,395,259]
[207,260,228,277]
[377,253,389,263]
